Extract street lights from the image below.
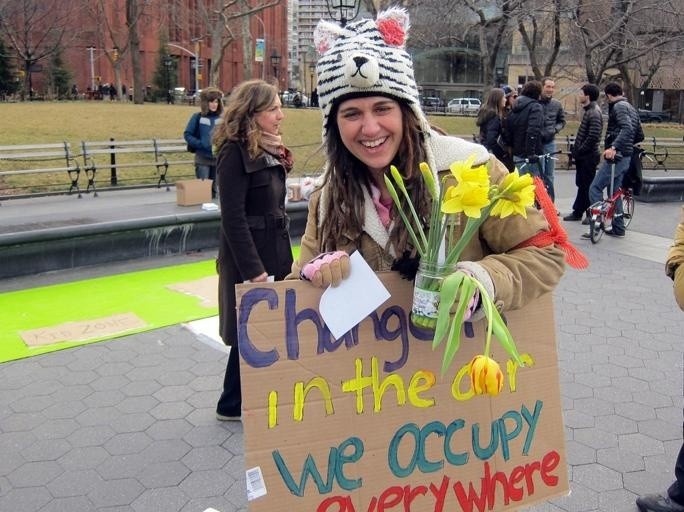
[324,0,362,26]
[165,39,199,96]
[271,50,281,79]
[308,59,315,107]
[640,91,644,108]
[516,85,522,95]
[495,67,504,87]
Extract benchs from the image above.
[0,141,84,199]
[79,136,197,196]
[555,133,684,172]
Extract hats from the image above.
[313,5,431,143]
[503,85,518,97]
[200,88,223,117]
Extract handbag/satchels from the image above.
[188,130,201,153]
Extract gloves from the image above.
[301,248,348,282]
[456,267,480,322]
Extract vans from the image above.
[420,95,482,113]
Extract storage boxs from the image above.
[175,178,213,205]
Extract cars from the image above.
[171,82,313,107]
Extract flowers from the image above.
[381,157,533,395]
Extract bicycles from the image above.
[582,149,654,245]
[513,148,562,210]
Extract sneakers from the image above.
[636,493,684,512]
[563,213,625,239]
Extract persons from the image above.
[479,72,646,240]
[0,79,196,105]
[210,77,296,424]
[278,87,319,108]
[183,85,224,199]
[632,202,684,512]
[283,6,567,327]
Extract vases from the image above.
[408,259,458,332]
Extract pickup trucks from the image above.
[637,107,672,124]
